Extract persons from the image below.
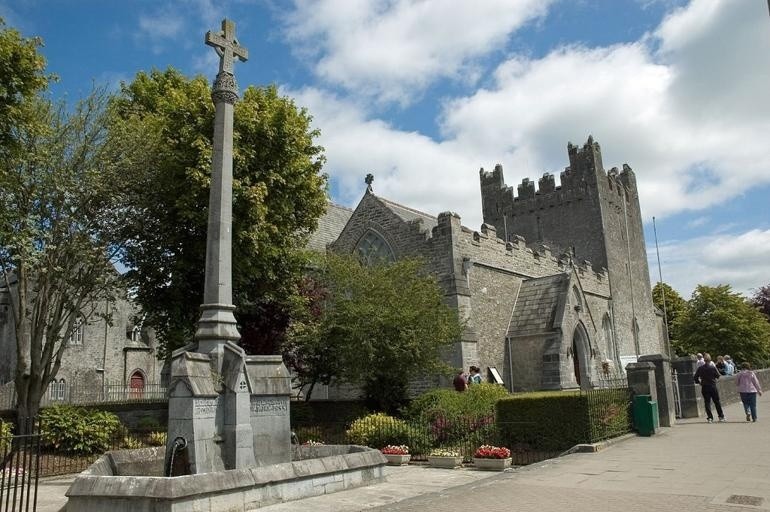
[453,369,469,392]
[696,352,737,376]
[694,353,726,422]
[467,366,483,384]
[734,362,762,422]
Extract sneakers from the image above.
[708,416,726,423]
[747,412,751,421]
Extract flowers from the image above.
[429,449,458,456]
[382,445,409,454]
[475,445,510,458]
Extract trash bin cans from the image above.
[634,395,657,436]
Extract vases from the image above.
[427,456,464,469]
[384,454,411,467]
[473,457,511,471]
